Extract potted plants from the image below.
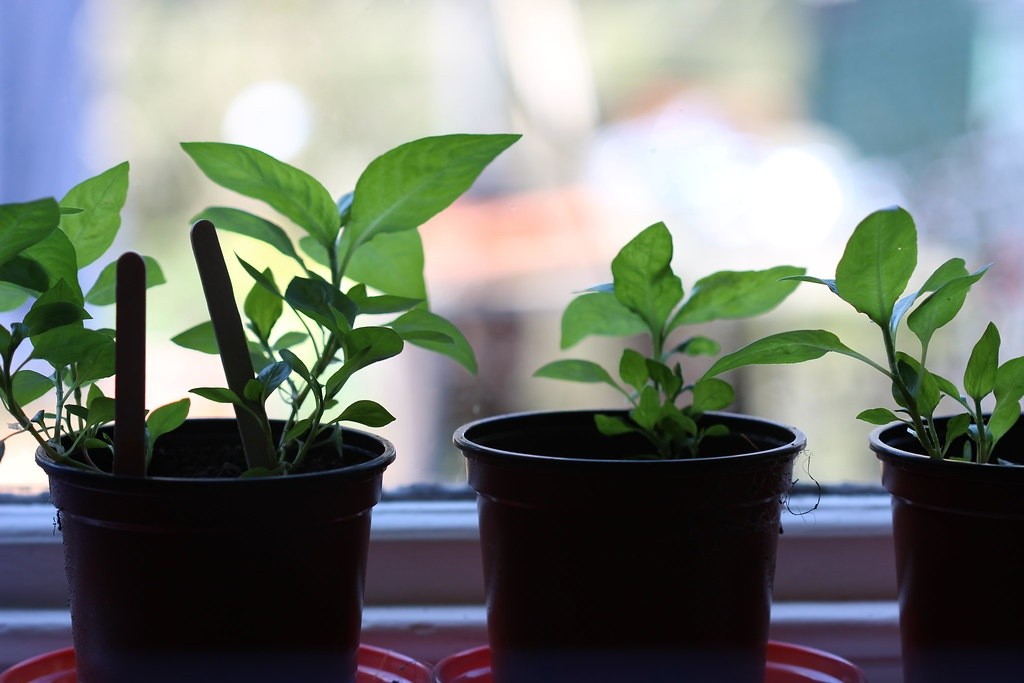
[696,201,1023,683]
[0,130,526,683]
[453,220,807,683]
